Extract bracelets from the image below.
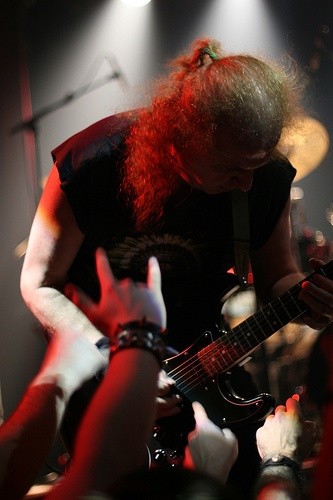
[108,321,166,362]
[256,455,301,473]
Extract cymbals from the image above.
[272,115,331,184]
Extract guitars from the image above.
[62,259,333,500]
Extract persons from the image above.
[254,398,312,500]
[180,400,240,500]
[1,248,166,500]
[2,316,104,499]
[20,37,333,394]
